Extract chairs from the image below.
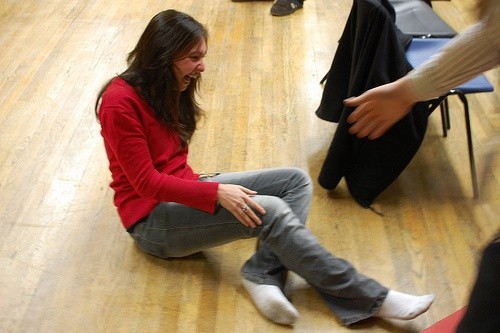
[315,0,494,215]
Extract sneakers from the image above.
[271,0,303,16]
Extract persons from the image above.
[343,0,500,333]
[96,9,435,326]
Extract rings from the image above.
[241,205,248,212]
